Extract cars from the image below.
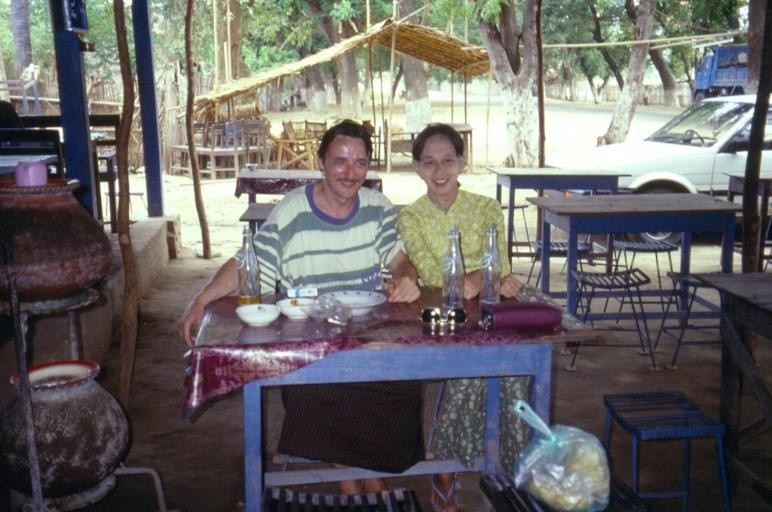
[532,95,772,247]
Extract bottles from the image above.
[441,224,466,309]
[480,223,500,304]
[238,226,260,306]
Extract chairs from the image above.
[180,116,385,180]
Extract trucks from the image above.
[691,46,747,106]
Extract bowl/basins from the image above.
[275,297,320,319]
[235,303,281,328]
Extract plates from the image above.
[320,290,387,316]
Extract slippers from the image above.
[430,472,457,512]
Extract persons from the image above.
[396,122,534,512]
[175,118,426,497]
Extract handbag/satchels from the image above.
[480,303,561,330]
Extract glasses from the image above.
[419,307,468,327]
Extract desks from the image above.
[235,169,411,233]
[691,269,772,511]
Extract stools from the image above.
[603,390,732,512]
[486,166,771,375]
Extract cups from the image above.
[16,159,48,188]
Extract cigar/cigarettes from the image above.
[181,346,194,359]
